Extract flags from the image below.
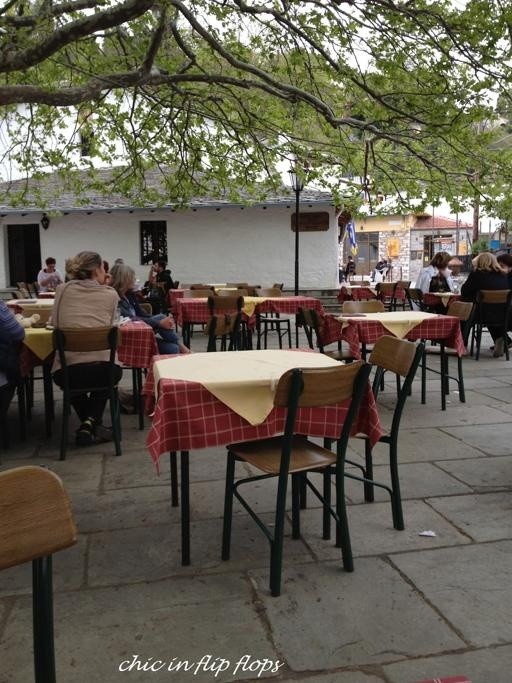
[343,217,360,253]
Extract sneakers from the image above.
[75,417,96,444]
[95,425,114,441]
[490,335,512,350]
[493,337,504,358]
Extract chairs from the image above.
[346,264,355,281]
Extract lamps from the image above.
[40,212,50,230]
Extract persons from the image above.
[411,252,459,315]
[370,258,396,282]
[0,299,27,450]
[52,248,122,448]
[339,255,357,282]
[489,254,512,350]
[114,257,125,264]
[107,261,193,357]
[37,256,63,292]
[102,259,110,272]
[458,252,507,359]
[139,251,173,317]
